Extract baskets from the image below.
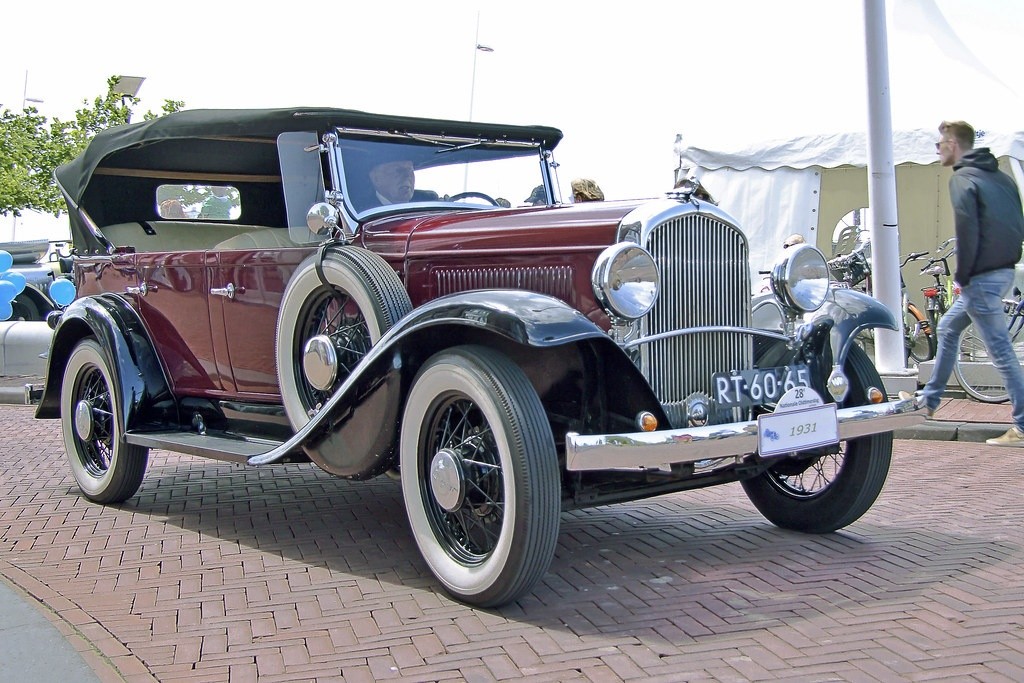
[828,251,871,287]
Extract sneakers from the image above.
[898,391,934,420]
[986,427,1024,447]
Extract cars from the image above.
[0,107,932,607]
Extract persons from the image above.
[524,179,604,206]
[898,121,1024,448]
[353,156,439,213]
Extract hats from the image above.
[524,185,545,202]
[496,198,510,208]
[368,147,423,171]
[674,176,700,194]
[571,179,604,201]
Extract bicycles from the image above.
[755,238,1024,405]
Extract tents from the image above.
[675,1,1023,361]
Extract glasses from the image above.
[935,141,956,149]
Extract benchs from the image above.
[213,227,319,248]
[99,221,272,252]
